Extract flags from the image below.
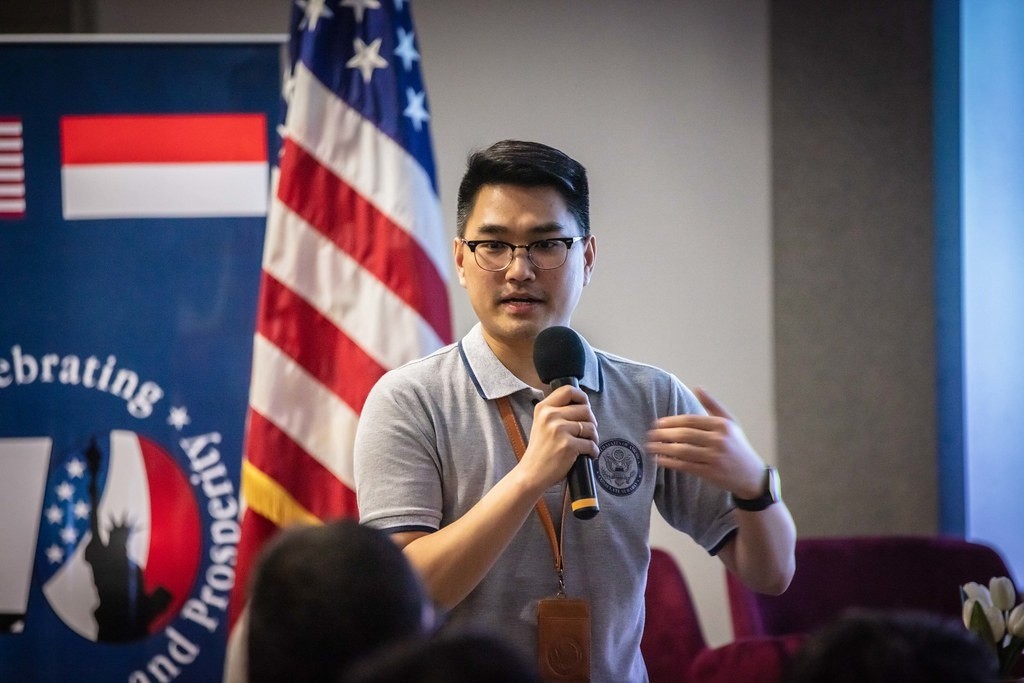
[223,0,456,683]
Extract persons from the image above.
[355,139,797,683]
[246,523,423,683]
[348,633,535,683]
[789,613,1000,683]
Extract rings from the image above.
[577,421,583,437]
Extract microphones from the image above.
[533,325,599,520]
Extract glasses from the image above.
[463,236,584,273]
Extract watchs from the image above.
[731,465,782,511]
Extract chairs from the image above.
[641,533,1017,683]
